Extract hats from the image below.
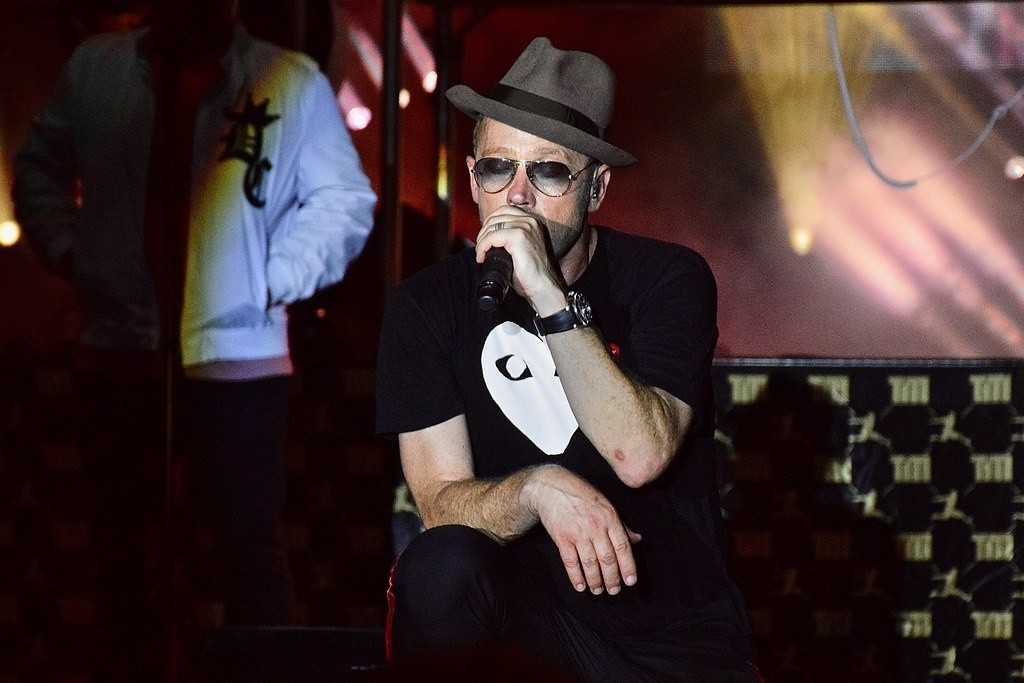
[445,38,638,167]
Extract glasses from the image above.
[470,157,597,197]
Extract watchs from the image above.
[534,286,595,336]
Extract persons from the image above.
[122,1,380,636]
[375,36,762,683]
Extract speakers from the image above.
[196,622,389,683]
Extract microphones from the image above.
[474,244,514,315]
